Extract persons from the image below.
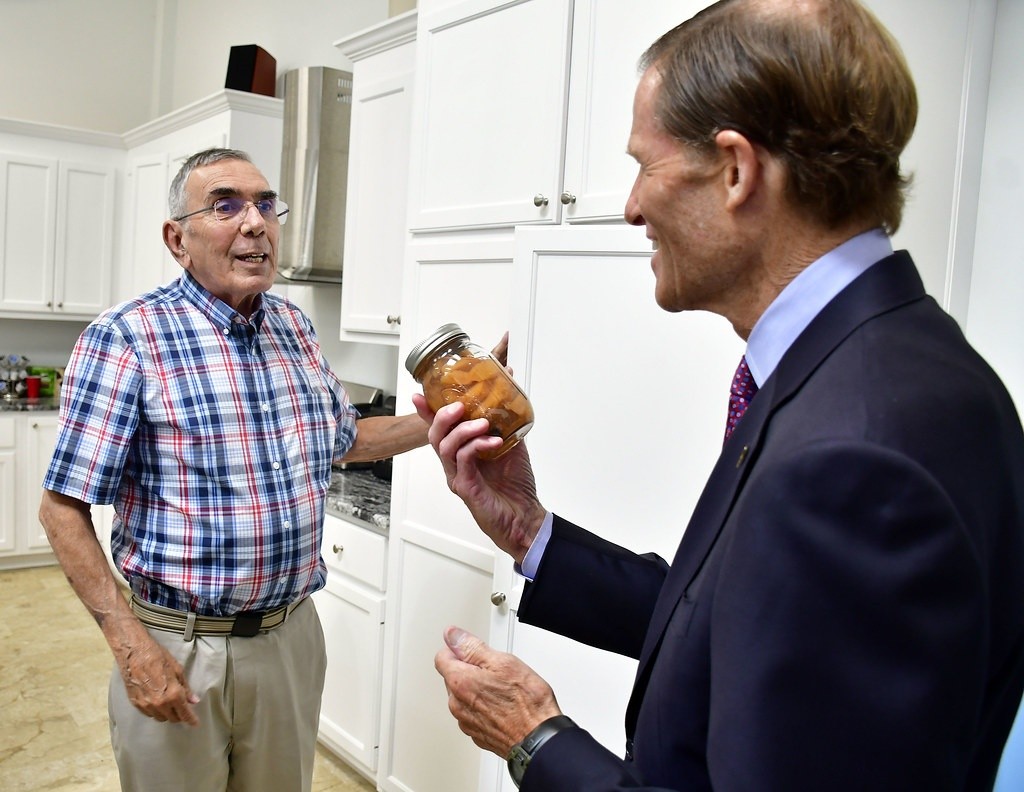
[411,0,1024,792]
[38,150,513,792]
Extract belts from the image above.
[132,595,306,638]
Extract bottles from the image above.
[401,323,535,460]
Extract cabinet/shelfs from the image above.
[314,0,744,792]
[0,89,285,319]
[0,411,129,589]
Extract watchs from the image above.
[507,715,580,789]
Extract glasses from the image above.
[175,199,288,224]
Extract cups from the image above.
[26,376,41,404]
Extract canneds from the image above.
[404,323,536,461]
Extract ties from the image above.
[724,356,757,444]
